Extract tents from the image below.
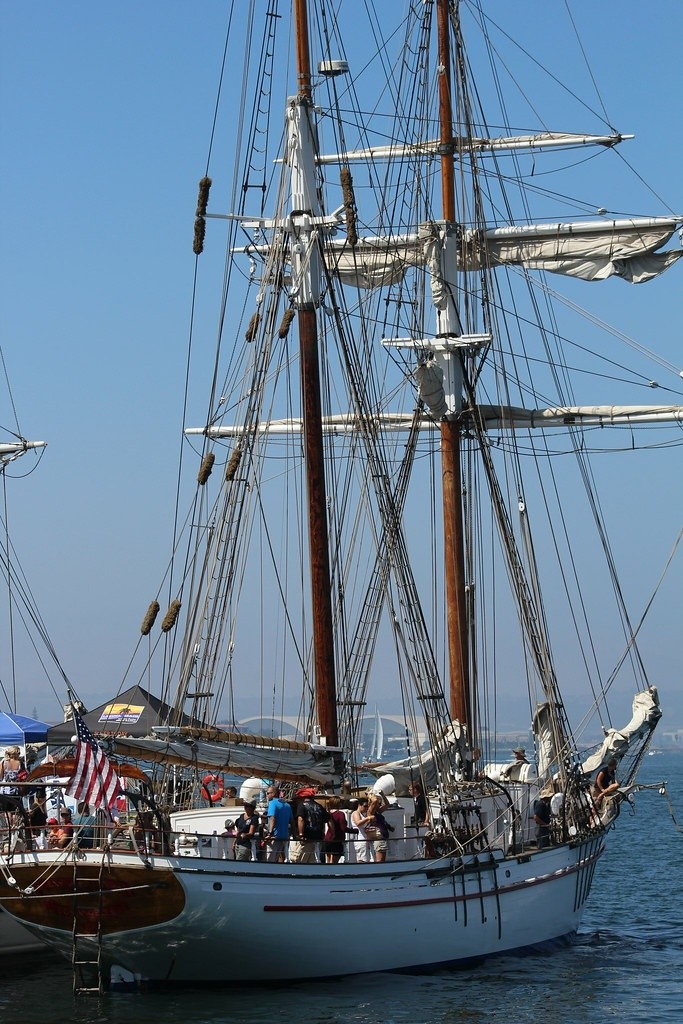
[0,685,223,805]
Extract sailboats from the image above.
[0,2,681,1000]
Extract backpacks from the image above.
[17,756,28,795]
[303,802,324,840]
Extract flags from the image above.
[65,700,121,813]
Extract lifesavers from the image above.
[199,773,226,802]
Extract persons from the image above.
[218,786,395,864]
[512,747,530,764]
[30,790,98,848]
[408,781,431,857]
[586,759,619,827]
[534,789,555,848]
[0,745,25,795]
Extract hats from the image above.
[48,818,58,825]
[245,798,256,808]
[225,819,235,828]
[296,788,318,797]
[539,790,555,799]
[512,747,526,757]
[61,808,70,814]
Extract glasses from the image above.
[408,789,413,791]
[266,792,273,794]
[360,805,368,807]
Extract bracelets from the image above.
[368,816,371,820]
